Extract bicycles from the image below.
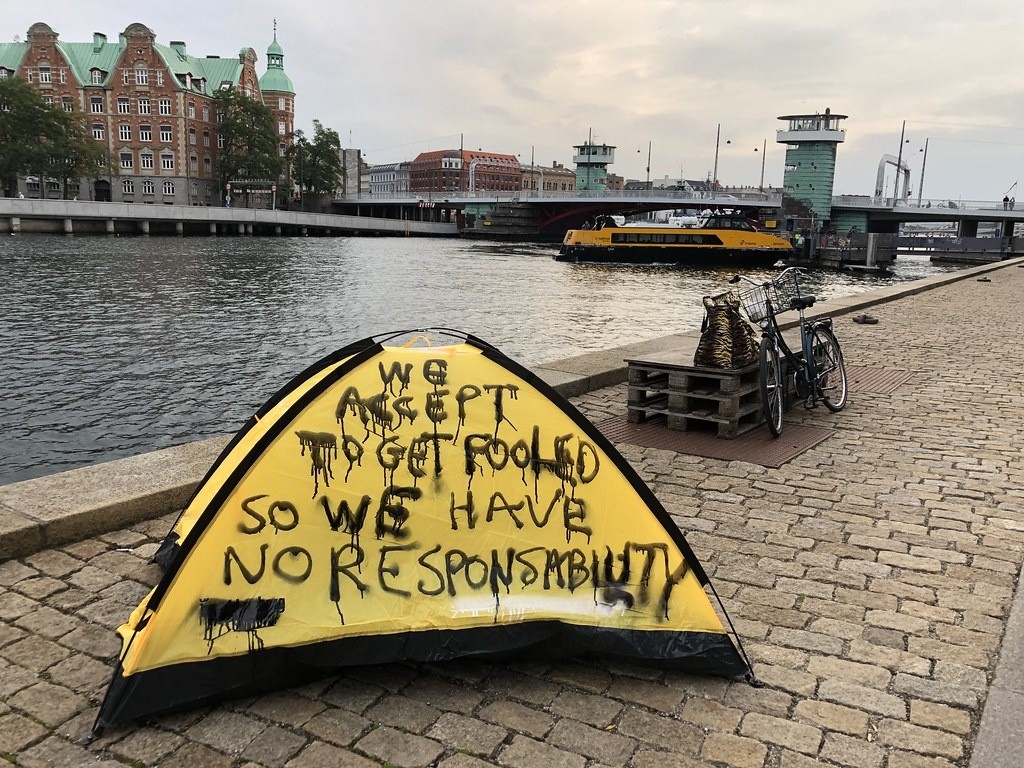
[728,266,848,437]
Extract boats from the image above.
[552,214,794,266]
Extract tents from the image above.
[87,326,756,746]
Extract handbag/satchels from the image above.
[694,291,761,370]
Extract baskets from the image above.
[738,277,797,322]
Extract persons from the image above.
[802,227,837,238]
[582,220,591,230]
[73,196,77,201]
[847,227,855,238]
[17,191,24,199]
[1009,196,1015,211]
[369,189,373,199]
[1003,195,1009,211]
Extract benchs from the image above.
[623,332,830,439]
